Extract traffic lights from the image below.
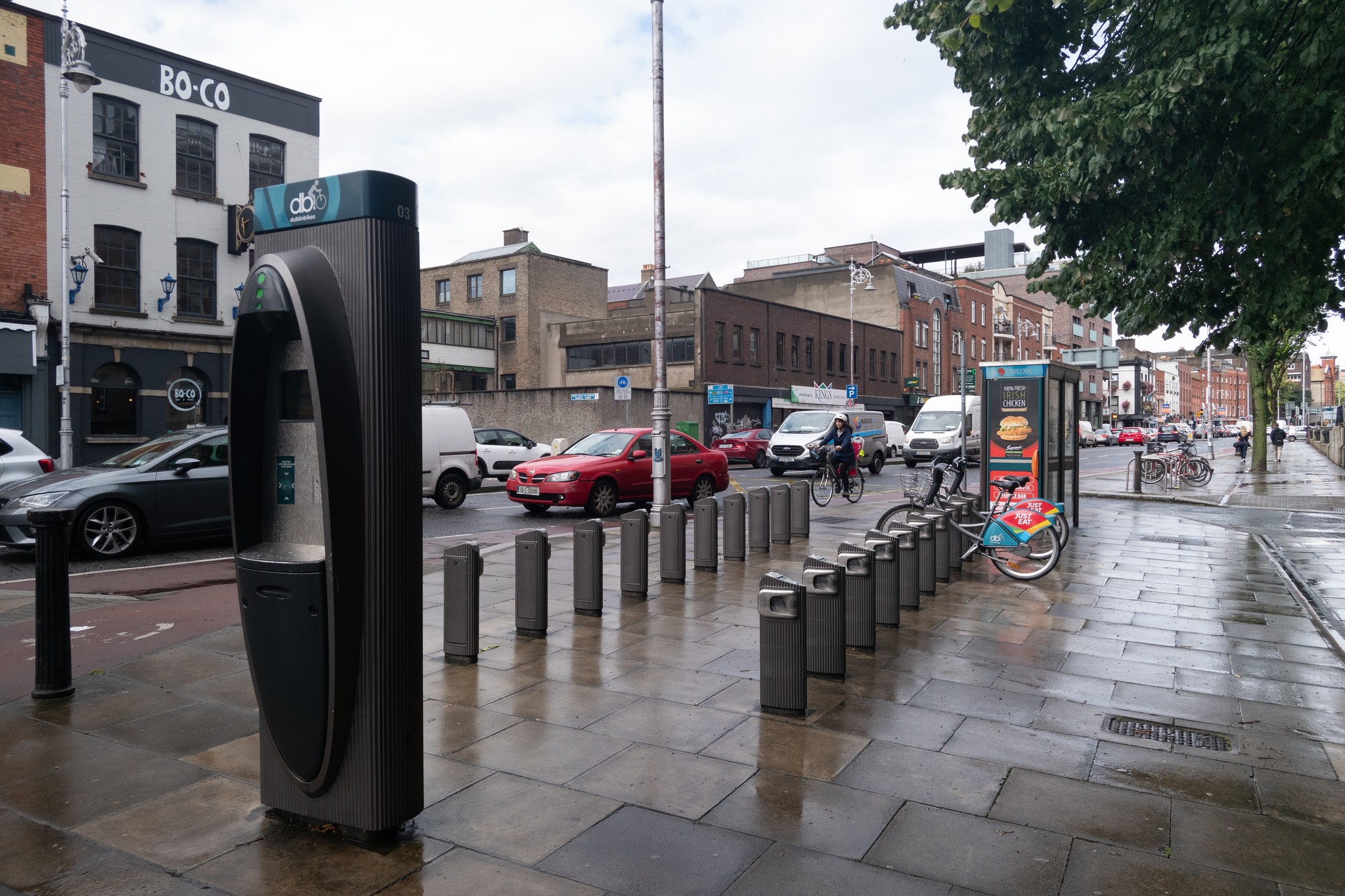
[1113,413,1117,420]
[1200,408,1204,416]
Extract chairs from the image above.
[994,323,1000,334]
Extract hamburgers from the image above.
[997,416,1032,441]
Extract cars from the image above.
[1079,419,1321,449]
[0,426,57,487]
[709,428,776,468]
[884,420,907,459]
[505,427,730,518]
[0,423,230,561]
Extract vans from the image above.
[765,410,889,477]
[902,394,980,467]
[422,399,481,511]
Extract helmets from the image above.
[835,414,847,423]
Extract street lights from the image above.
[1109,347,1125,430]
[51,0,102,472]
[844,253,877,411]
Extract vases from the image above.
[1124,386,1128,390]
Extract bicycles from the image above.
[875,454,1069,582]
[806,445,866,507]
[1141,437,1215,487]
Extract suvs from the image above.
[472,427,552,485]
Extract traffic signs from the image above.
[707,384,734,404]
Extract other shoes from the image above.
[1275,458,1281,462]
[831,479,836,484]
[842,491,850,497]
[1241,459,1246,463]
[1245,442,1251,447]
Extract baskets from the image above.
[808,447,828,463]
[924,464,954,487]
[899,468,932,497]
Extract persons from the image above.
[1306,424,1311,438]
[1236,425,1252,462]
[1270,423,1287,462]
[814,413,855,498]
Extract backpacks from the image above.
[1273,431,1283,444]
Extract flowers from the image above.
[1140,380,1155,398]
[1122,381,1131,391]
[1121,398,1131,414]
[1102,393,1108,408]
[1107,384,1119,397]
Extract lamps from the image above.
[67,261,90,305]
[157,272,179,312]
[232,281,245,320]
[1175,364,1179,370]
